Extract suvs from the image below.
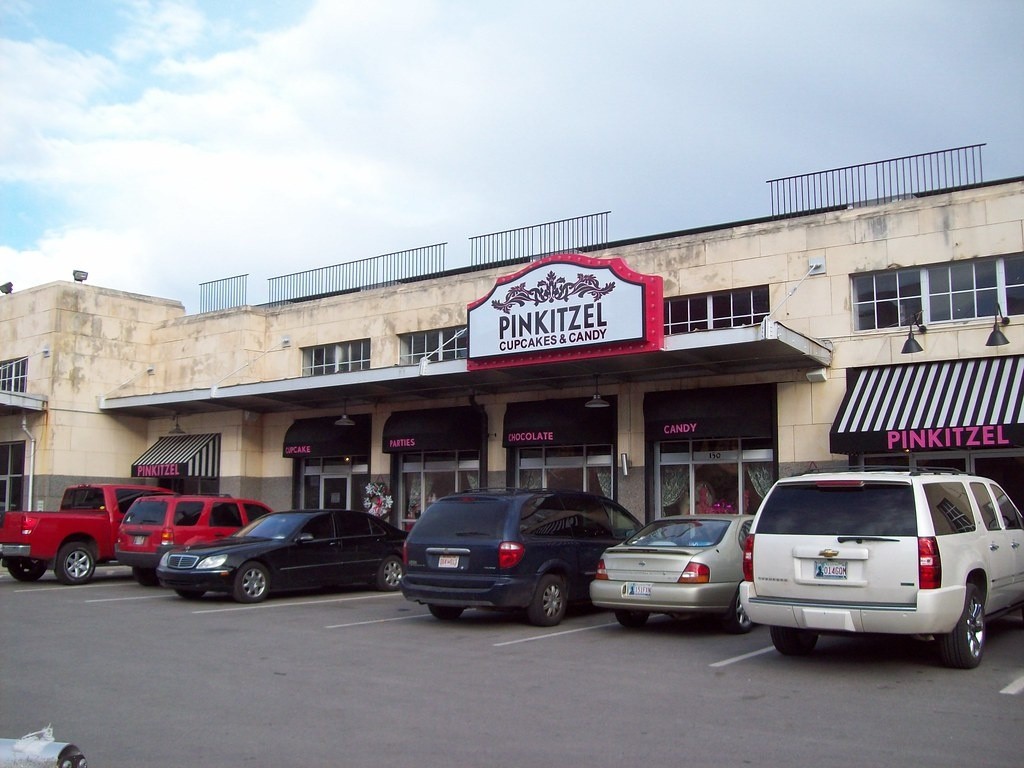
[399,488,645,628]
[739,465,1024,669]
[115,492,274,587]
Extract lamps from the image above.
[986,303,1010,346]
[335,398,355,425]
[585,372,610,407]
[169,415,186,435]
[620,453,632,475]
[900,312,927,353]
[0,281,13,294]
[73,270,88,282]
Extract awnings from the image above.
[829,355,1024,454]
[382,408,482,452]
[283,415,369,457]
[502,398,612,446]
[644,392,773,443]
[132,434,219,478]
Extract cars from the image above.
[157,510,410,604]
[590,514,755,633]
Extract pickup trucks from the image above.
[0,484,179,586]
